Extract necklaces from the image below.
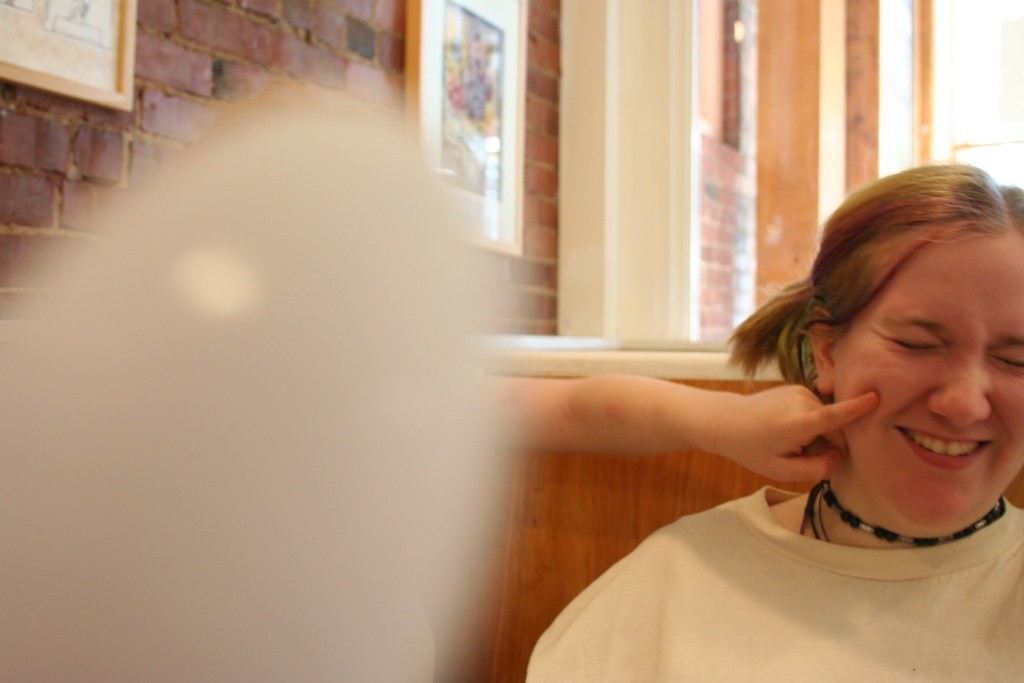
[800,478,1003,547]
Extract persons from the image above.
[525,160,1024,683]
[488,373,881,483]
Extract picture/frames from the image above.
[405,0,529,256]
[0,0,139,114]
